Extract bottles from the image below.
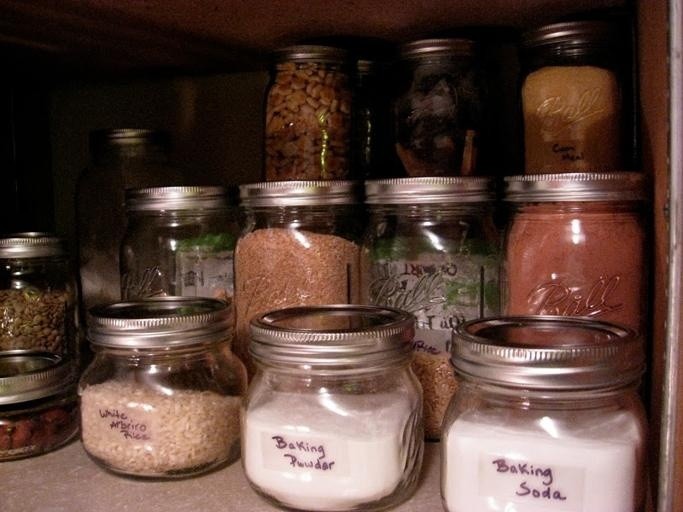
[240,305,426,510]
[234,179,362,337]
[73,128,166,309]
[499,176,649,329]
[403,38,476,177]
[519,21,631,175]
[0,232,73,356]
[78,300,246,483]
[118,185,243,306]
[266,47,384,174]
[363,177,504,445]
[0,352,85,464]
[441,317,654,512]
